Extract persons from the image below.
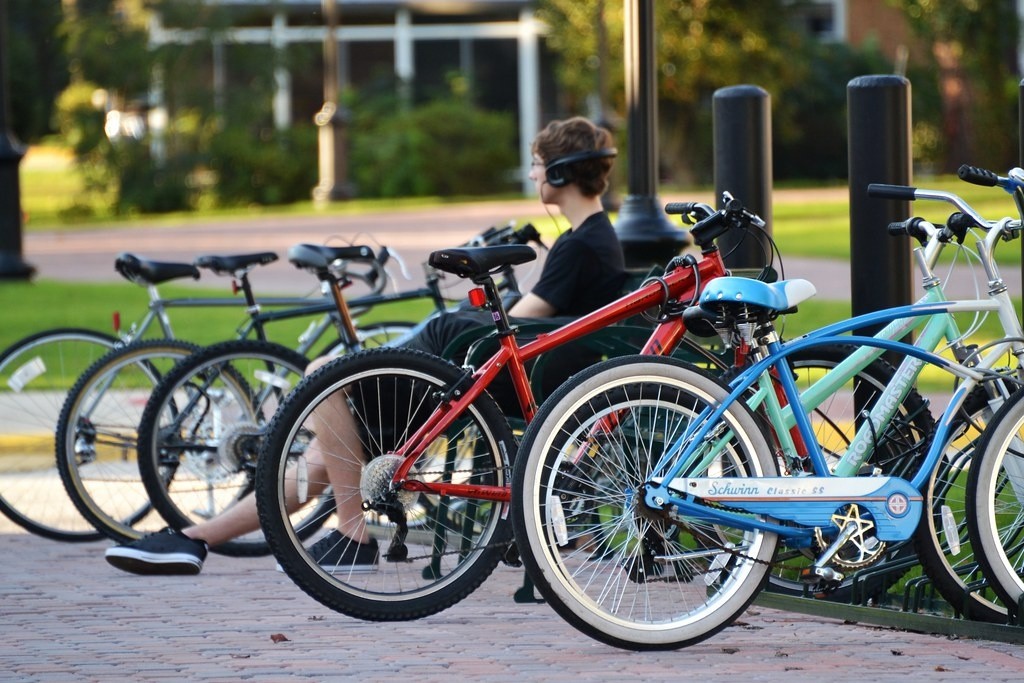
[105,116,623,575]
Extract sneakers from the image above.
[105,527,210,575]
[275,528,379,576]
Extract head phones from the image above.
[544,147,618,187]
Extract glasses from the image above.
[530,158,545,170]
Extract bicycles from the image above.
[256,188,949,624]
[0,222,560,559]
[507,161,1024,654]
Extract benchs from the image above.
[421,266,780,604]
